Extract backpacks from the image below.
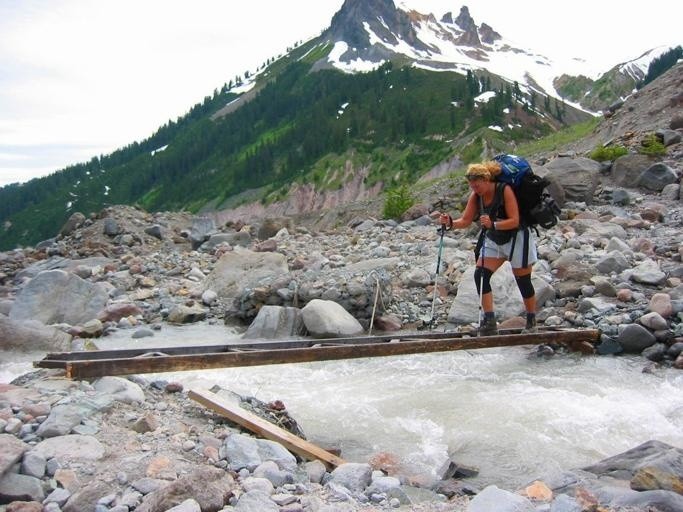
[481,153,563,232]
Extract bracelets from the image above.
[491,220,496,231]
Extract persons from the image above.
[439,160,537,334]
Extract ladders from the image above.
[33,324,599,380]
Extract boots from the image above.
[517,312,539,336]
[464,310,499,334]
